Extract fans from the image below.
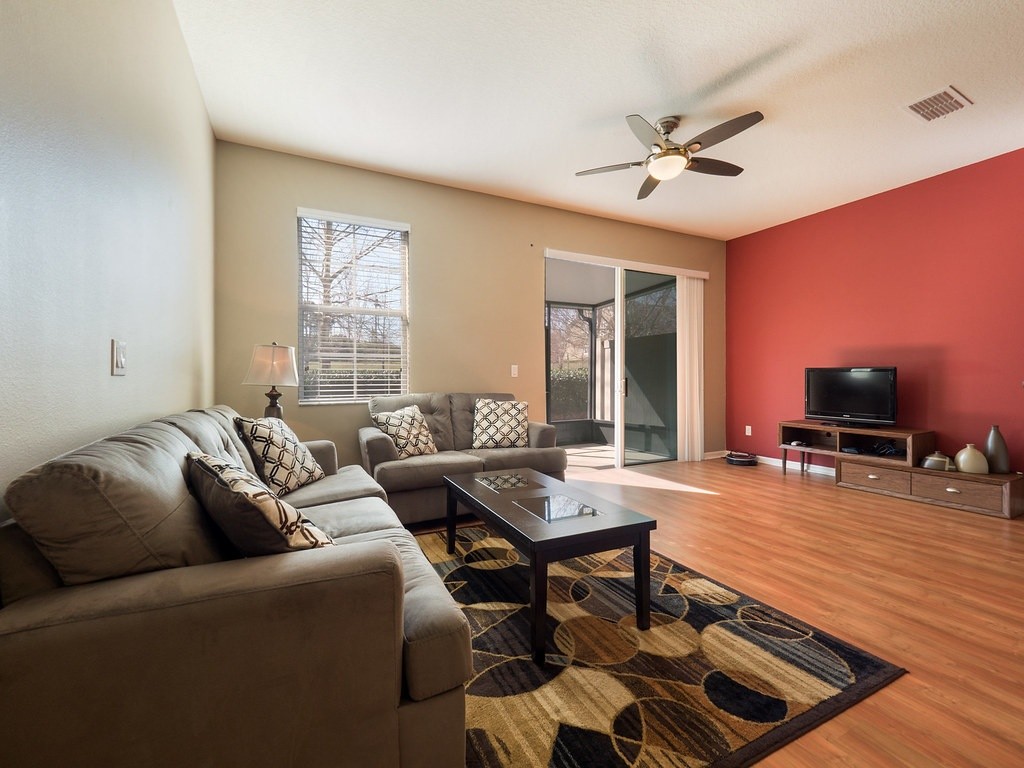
[575,110,764,200]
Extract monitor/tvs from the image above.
[805,366,897,429]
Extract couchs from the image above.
[358,392,567,523]
[0,405,473,768]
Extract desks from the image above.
[442,467,656,664]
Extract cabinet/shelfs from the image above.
[777,420,1024,519]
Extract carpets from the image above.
[411,519,909,768]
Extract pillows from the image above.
[186,451,338,552]
[233,417,325,496]
[477,475,528,490]
[370,404,438,460]
[472,398,528,448]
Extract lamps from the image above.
[645,150,689,183]
[241,342,300,420]
